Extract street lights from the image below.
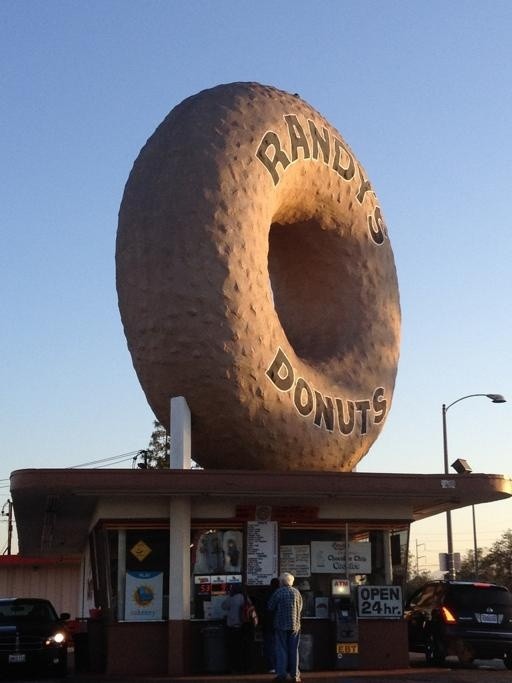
[440,392,508,581]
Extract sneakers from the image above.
[273,675,301,683]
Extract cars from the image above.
[0,596,71,683]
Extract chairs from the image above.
[27,607,51,619]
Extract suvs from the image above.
[403,579,512,671]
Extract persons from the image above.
[258,577,280,674]
[221,583,253,677]
[266,571,303,682]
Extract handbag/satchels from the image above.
[240,602,265,648]
